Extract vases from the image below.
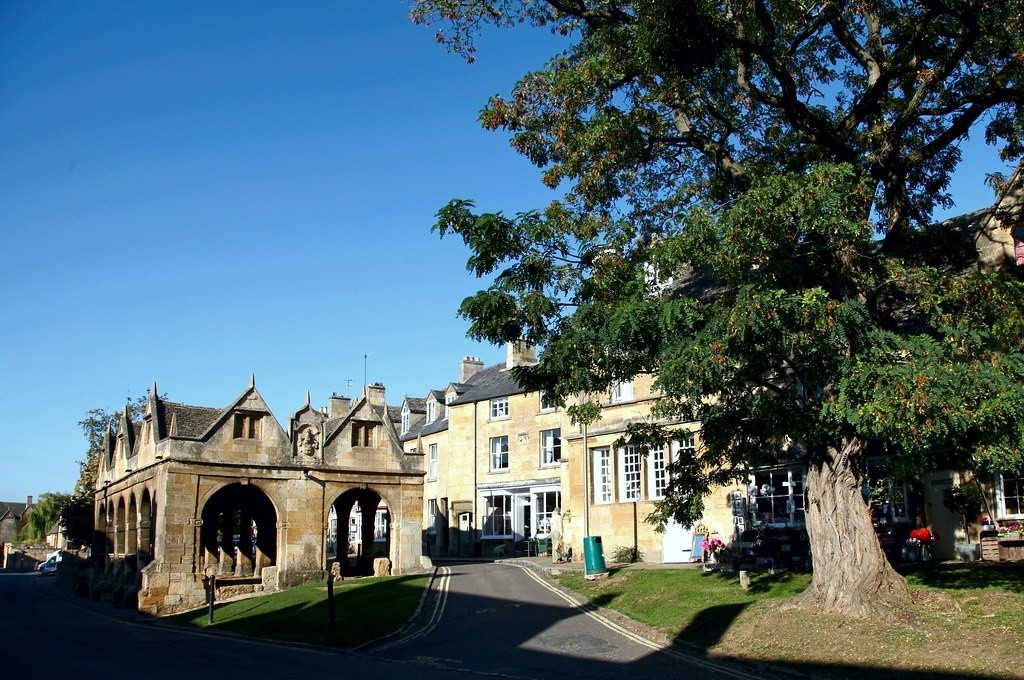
[1004,533,1021,540]
[983,525,994,531]
[710,551,718,562]
[917,543,936,566]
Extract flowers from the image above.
[699,538,725,552]
[905,526,939,561]
[998,523,1024,536]
[978,516,994,526]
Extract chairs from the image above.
[494,544,507,558]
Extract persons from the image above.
[33,561,39,570]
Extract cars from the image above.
[37,550,63,576]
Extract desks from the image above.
[515,540,537,557]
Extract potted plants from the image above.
[942,481,986,515]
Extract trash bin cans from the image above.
[583,536,608,575]
